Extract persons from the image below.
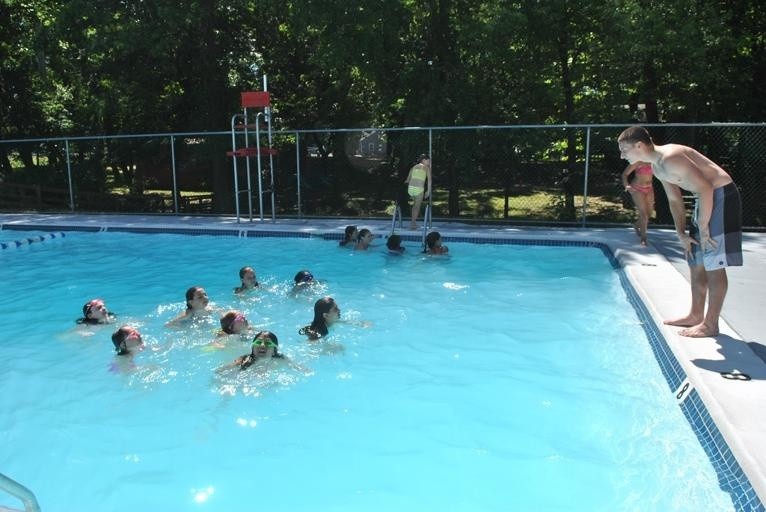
[286,269,325,294]
[355,228,373,250]
[420,231,449,256]
[404,153,432,229]
[339,225,358,247]
[621,160,656,248]
[105,324,160,375]
[231,266,263,296]
[211,330,312,378]
[617,126,744,338]
[384,234,407,257]
[75,298,117,325]
[298,295,342,340]
[165,286,224,329]
[212,309,261,349]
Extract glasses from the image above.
[252,340,275,348]
[301,275,313,281]
[127,328,138,338]
[91,299,102,306]
[233,315,243,322]
